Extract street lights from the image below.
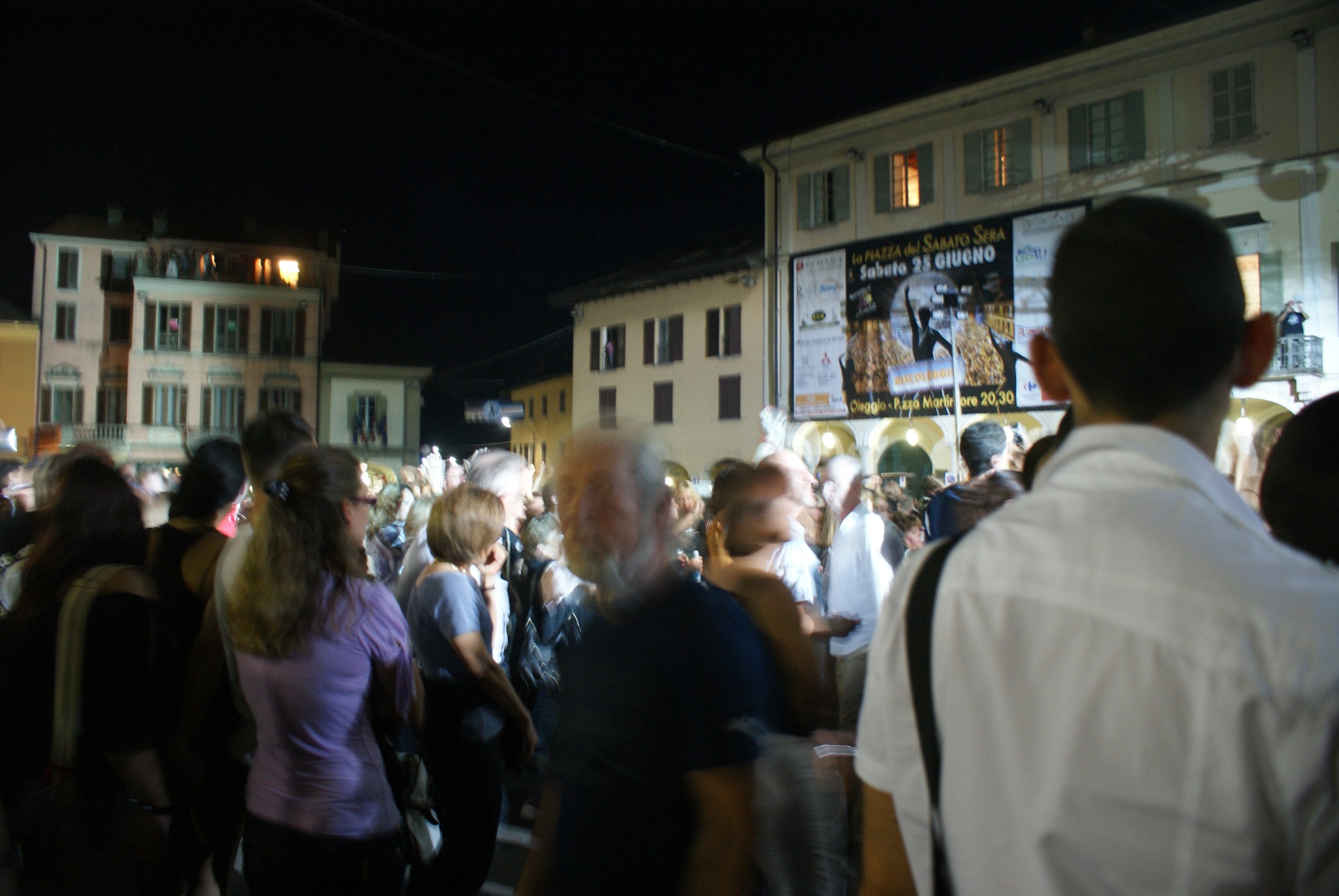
[929,283,974,483]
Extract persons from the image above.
[855,195,1339,896]
[4,386,1339,895]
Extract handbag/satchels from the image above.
[506,558,586,697]
[29,759,155,891]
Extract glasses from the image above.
[346,494,379,508]
